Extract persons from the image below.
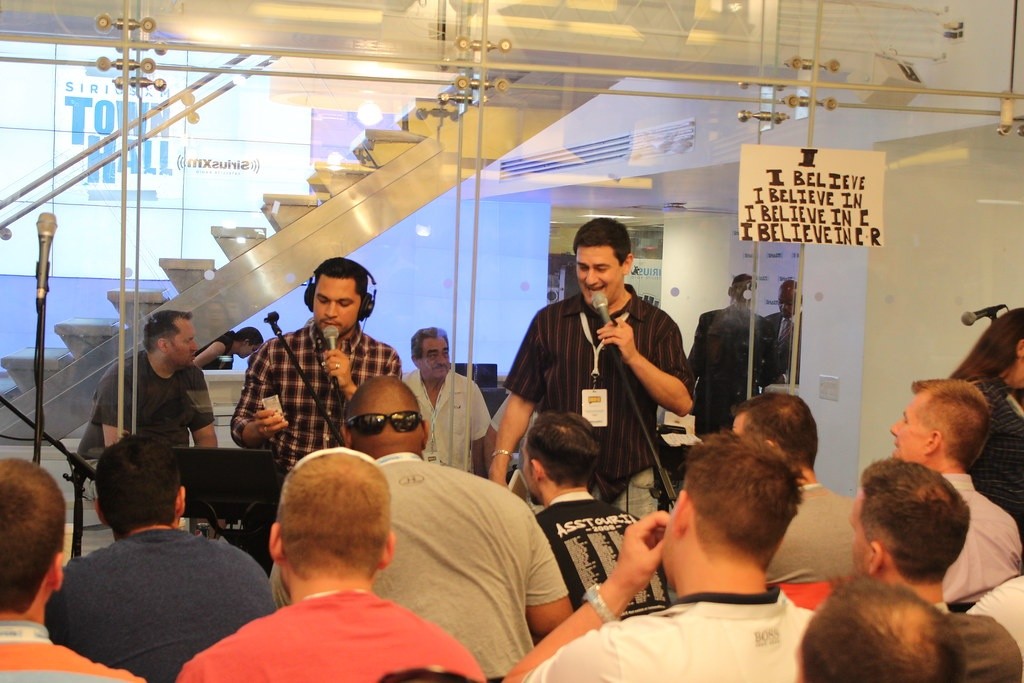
[229,257,403,478]
[402,327,492,481]
[44,433,276,683]
[485,217,697,520]
[948,308,1024,577]
[192,326,264,370]
[732,392,854,585]
[174,452,487,683]
[269,376,573,679]
[484,391,512,479]
[890,378,1024,603]
[0,457,148,683]
[76,309,218,532]
[503,432,816,683]
[850,459,1024,683]
[764,280,802,384]
[797,581,967,683]
[522,412,672,622]
[687,274,775,437]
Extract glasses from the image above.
[346,411,425,431]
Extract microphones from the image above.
[324,326,339,389]
[960,304,1007,326]
[36,212,58,312]
[591,291,613,326]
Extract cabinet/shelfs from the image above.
[624,214,729,358]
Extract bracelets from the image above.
[491,449,513,460]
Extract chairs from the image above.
[453,361,498,387]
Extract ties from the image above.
[778,318,790,346]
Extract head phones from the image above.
[304,257,378,322]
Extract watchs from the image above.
[582,582,620,624]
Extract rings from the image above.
[335,363,340,368]
